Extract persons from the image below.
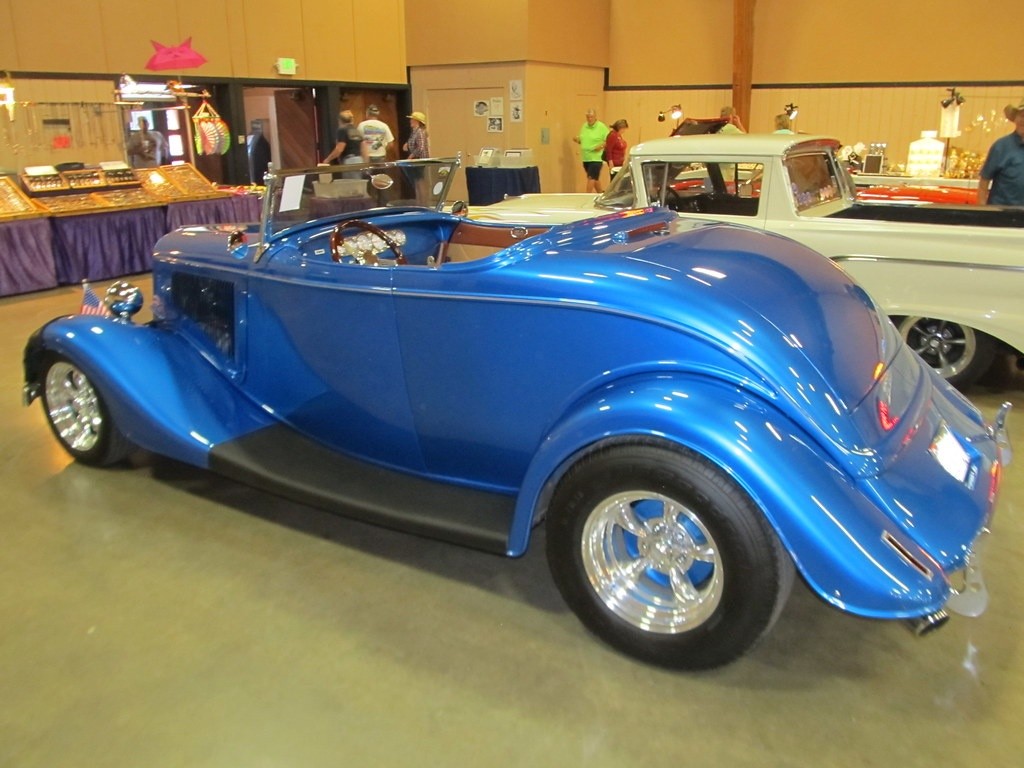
[246,120,271,186]
[125,116,168,169]
[402,111,431,208]
[356,104,395,206]
[704,106,747,192]
[605,118,629,182]
[322,109,364,179]
[976,97,1024,205]
[771,114,796,134]
[572,109,610,193]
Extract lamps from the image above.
[658,104,682,131]
[783,102,797,134]
[0,69,17,121]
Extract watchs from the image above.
[600,144,604,149]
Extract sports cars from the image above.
[22,153,1003,673]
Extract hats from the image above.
[406,111,425,124]
[1004,100,1023,121]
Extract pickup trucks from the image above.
[420,130,1023,392]
